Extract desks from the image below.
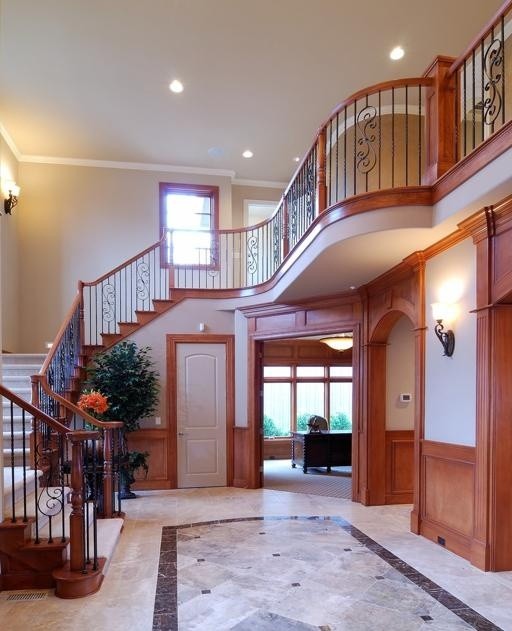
[290,430,352,474]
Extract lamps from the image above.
[318,337,353,352]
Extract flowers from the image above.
[75,388,114,431]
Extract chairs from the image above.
[308,415,328,431]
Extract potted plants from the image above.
[71,336,163,480]
